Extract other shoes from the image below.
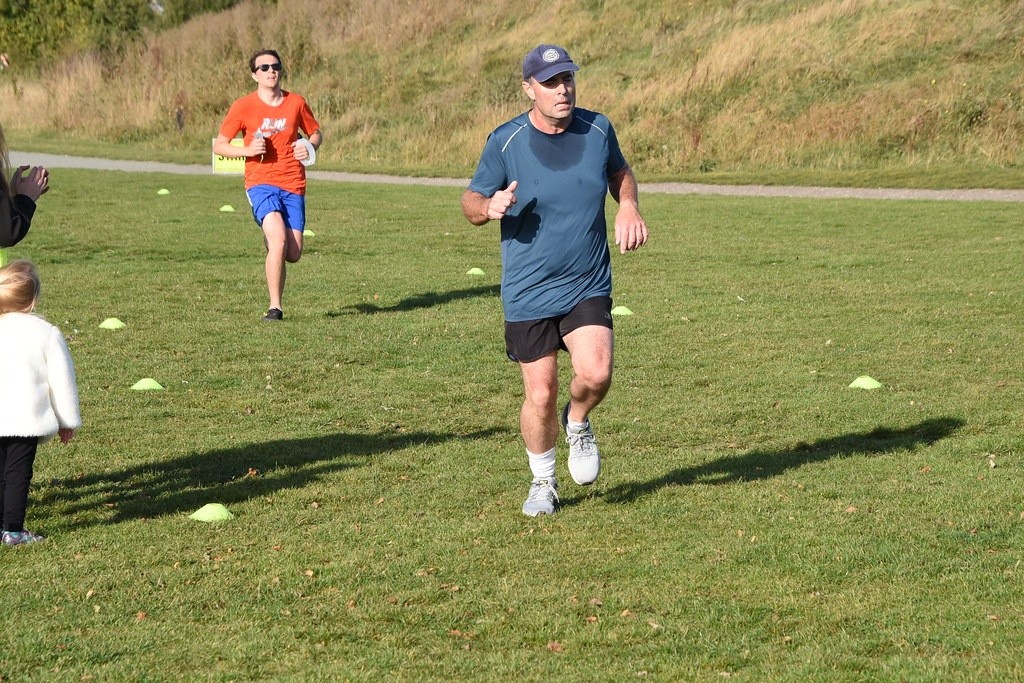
[266,306,284,319]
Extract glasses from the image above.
[255,63,282,72]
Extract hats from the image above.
[522,44,580,83]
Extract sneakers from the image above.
[561,401,599,486]
[521,477,560,517]
[0,530,44,545]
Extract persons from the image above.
[0,124,50,270]
[461,45,649,517]
[213,49,323,320]
[0,260,82,545]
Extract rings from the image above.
[300,153,303,157]
